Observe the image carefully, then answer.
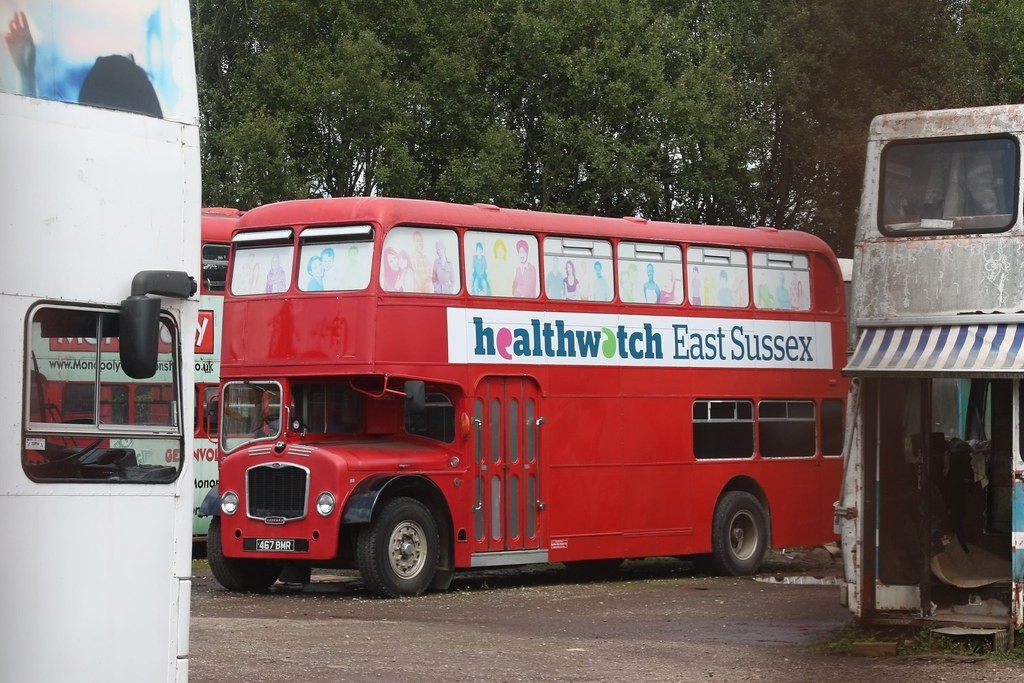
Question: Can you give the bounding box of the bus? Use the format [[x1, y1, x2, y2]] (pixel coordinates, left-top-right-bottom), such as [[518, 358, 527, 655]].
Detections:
[[21, 201, 334, 545], [195, 195, 853, 599], [835, 105, 1024, 638], [0, 2, 207, 683]]
